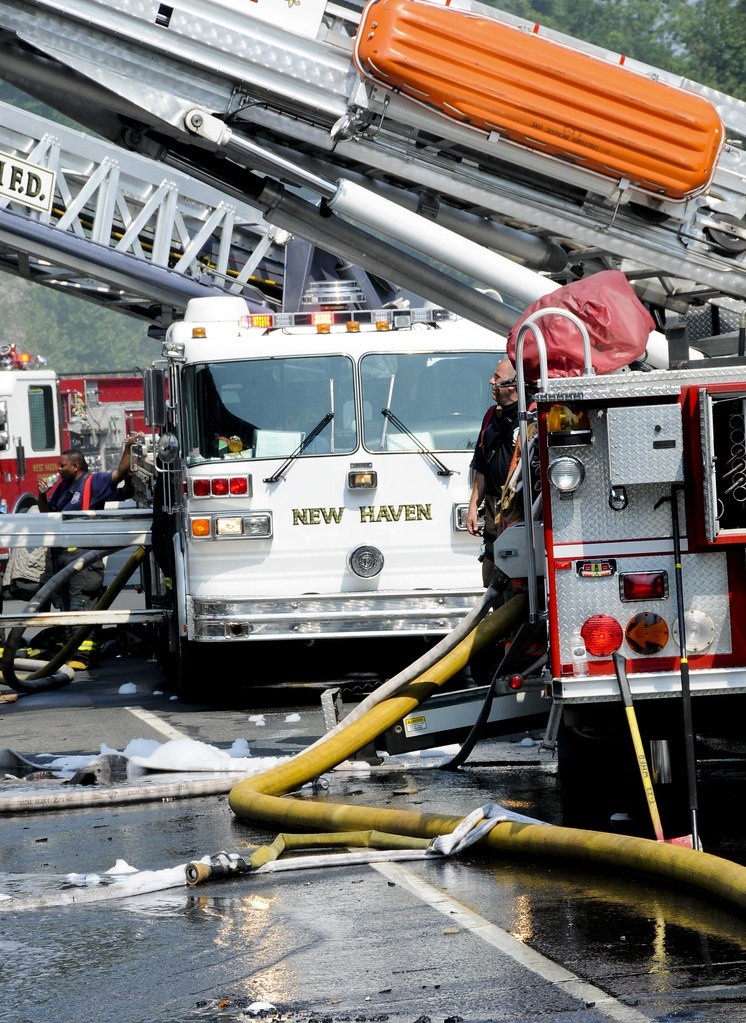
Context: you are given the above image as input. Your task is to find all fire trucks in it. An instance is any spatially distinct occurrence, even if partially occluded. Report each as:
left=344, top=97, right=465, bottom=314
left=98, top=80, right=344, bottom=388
left=146, top=295, right=520, bottom=689
left=0, top=368, right=160, bottom=515
left=514, top=302, right=746, bottom=826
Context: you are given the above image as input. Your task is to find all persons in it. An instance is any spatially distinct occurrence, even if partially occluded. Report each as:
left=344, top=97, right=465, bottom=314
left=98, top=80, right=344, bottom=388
left=35, top=430, right=143, bottom=665
left=465, top=357, right=537, bottom=613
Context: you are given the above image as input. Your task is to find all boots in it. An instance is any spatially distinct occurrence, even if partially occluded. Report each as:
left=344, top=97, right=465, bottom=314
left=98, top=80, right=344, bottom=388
left=65, top=586, right=107, bottom=673
